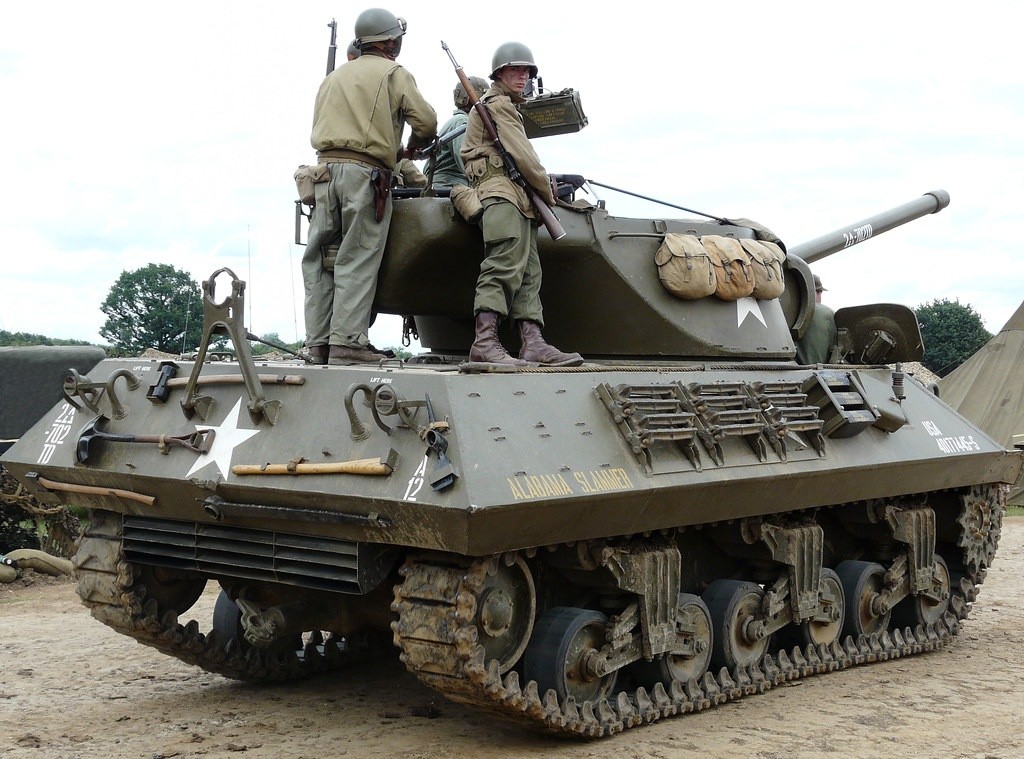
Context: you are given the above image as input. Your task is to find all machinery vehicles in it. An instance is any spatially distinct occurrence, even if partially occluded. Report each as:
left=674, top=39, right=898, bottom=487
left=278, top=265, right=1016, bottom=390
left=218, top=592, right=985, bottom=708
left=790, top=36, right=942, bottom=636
left=0, top=172, right=1024, bottom=741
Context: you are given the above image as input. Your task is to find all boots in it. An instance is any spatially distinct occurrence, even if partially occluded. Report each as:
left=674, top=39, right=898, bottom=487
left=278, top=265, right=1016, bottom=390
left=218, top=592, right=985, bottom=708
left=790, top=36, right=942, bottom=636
left=518, top=318, right=584, bottom=368
left=470, top=310, right=528, bottom=367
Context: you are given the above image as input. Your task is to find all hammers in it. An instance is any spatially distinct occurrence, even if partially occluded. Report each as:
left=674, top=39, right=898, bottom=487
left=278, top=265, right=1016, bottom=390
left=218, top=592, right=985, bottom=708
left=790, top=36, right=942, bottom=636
left=153, top=363, right=307, bottom=407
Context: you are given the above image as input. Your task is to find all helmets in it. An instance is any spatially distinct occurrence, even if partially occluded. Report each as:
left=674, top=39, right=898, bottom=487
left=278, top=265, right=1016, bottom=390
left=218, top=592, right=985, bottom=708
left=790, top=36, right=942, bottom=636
left=454, top=76, right=491, bottom=112
left=346, top=39, right=361, bottom=59
left=489, top=42, right=538, bottom=79
left=353, top=8, right=407, bottom=49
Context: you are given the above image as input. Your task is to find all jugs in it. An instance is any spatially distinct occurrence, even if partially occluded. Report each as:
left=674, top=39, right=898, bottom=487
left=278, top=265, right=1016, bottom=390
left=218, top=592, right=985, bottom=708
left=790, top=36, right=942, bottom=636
left=294, top=165, right=314, bottom=205
left=449, top=183, right=483, bottom=223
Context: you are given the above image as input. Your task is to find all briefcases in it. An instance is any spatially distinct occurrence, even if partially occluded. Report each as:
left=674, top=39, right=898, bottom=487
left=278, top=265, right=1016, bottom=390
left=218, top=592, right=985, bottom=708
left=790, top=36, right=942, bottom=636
left=514, top=87, right=589, bottom=140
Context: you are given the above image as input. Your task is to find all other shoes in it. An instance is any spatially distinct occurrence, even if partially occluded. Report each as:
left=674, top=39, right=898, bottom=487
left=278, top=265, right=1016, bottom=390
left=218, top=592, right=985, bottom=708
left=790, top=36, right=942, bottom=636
left=304, top=346, right=329, bottom=364
left=328, top=345, right=388, bottom=366
left=367, top=340, right=398, bottom=358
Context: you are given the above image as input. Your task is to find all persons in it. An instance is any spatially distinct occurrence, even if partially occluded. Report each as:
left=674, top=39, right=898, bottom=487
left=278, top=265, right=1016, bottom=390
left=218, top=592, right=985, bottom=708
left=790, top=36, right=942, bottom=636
left=460, top=43, right=585, bottom=367
left=347, top=39, right=490, bottom=197
left=793, top=274, right=837, bottom=365
left=300, top=8, right=438, bottom=364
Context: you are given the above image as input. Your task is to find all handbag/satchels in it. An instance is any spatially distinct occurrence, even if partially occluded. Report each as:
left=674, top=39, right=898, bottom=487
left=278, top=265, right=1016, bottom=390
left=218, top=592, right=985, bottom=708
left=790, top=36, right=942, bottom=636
left=293, top=165, right=316, bottom=207
left=449, top=182, right=484, bottom=224
left=699, top=233, right=755, bottom=300
left=736, top=237, right=786, bottom=300
left=655, top=232, right=716, bottom=299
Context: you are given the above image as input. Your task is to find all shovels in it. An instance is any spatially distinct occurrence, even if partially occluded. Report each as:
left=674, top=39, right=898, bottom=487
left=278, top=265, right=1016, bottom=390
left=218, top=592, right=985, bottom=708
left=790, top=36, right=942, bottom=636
left=73, top=415, right=217, bottom=465
left=425, top=394, right=454, bottom=493
